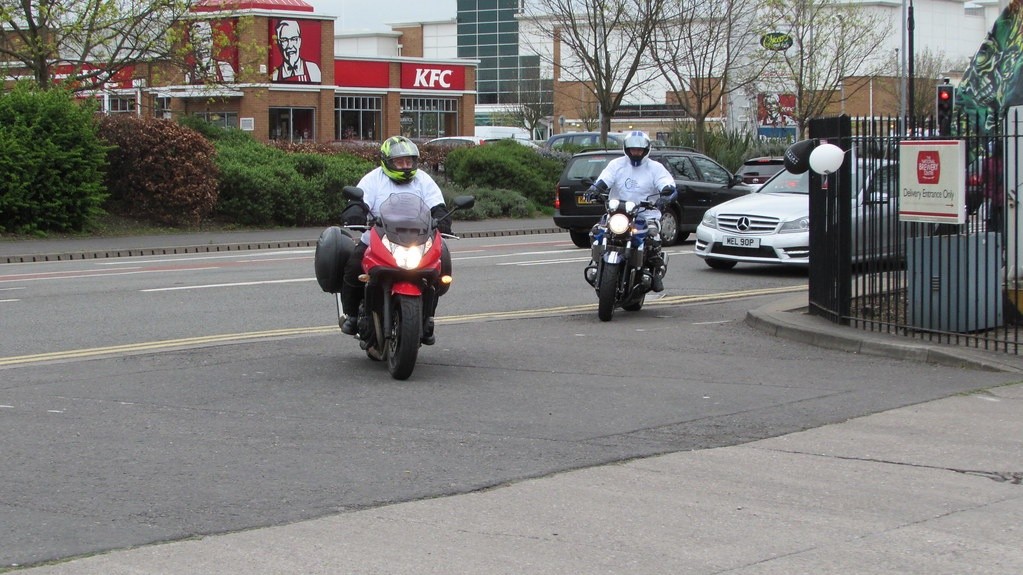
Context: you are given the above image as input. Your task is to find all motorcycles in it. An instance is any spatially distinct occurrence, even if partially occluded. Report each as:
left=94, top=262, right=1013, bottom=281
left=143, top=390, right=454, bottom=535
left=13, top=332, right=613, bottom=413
left=314, top=186, right=475, bottom=380
left=580, top=175, right=675, bottom=321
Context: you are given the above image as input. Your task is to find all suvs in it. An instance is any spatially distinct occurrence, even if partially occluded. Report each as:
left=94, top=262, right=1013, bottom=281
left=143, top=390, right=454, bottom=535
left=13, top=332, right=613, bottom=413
left=552, top=146, right=751, bottom=249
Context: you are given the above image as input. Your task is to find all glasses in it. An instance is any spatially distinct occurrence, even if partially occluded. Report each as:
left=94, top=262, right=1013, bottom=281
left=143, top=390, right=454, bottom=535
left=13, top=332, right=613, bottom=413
left=392, top=158, right=414, bottom=166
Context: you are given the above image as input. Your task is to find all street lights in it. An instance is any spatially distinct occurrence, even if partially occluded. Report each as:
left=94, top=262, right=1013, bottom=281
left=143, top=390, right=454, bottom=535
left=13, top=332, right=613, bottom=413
left=837, top=13, right=845, bottom=113
left=895, top=47, right=898, bottom=114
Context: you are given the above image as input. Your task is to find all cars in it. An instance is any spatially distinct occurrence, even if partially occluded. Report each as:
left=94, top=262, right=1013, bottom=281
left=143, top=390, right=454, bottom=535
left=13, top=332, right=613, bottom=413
left=695, top=154, right=958, bottom=271
left=734, top=154, right=809, bottom=187
left=544, top=134, right=663, bottom=149
left=967, top=157, right=987, bottom=207
left=425, top=138, right=540, bottom=152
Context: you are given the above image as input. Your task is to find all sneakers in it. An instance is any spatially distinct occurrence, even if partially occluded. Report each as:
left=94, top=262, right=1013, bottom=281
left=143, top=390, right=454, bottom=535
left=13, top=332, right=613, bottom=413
left=651, top=274, right=665, bottom=292
left=589, top=268, right=597, bottom=283
left=341, top=315, right=358, bottom=335
left=422, top=334, right=435, bottom=345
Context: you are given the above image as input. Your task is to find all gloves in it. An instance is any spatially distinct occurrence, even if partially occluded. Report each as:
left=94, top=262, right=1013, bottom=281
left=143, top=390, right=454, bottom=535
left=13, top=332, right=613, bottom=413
left=341, top=200, right=370, bottom=233
left=655, top=185, right=681, bottom=212
left=582, top=179, right=608, bottom=202
left=430, top=203, right=453, bottom=239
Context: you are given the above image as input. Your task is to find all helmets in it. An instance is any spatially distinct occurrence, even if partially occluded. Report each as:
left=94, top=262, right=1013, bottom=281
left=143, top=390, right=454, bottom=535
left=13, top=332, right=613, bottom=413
left=379, top=135, right=420, bottom=184
left=622, top=130, right=654, bottom=166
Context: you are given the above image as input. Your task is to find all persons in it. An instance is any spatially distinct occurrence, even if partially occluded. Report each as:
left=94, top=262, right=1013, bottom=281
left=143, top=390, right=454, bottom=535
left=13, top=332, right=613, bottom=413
left=583, top=131, right=677, bottom=293
left=400, top=124, right=415, bottom=137
left=271, top=128, right=276, bottom=139
left=277, top=127, right=288, bottom=138
left=341, top=136, right=452, bottom=345
left=342, top=126, right=357, bottom=139
left=295, top=128, right=311, bottom=139
left=984, top=139, right=1005, bottom=266
left=368, top=129, right=373, bottom=138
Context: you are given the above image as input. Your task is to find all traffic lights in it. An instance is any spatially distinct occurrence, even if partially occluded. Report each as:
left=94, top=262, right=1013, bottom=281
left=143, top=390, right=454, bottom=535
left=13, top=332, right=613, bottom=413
left=937, top=85, right=954, bottom=130
